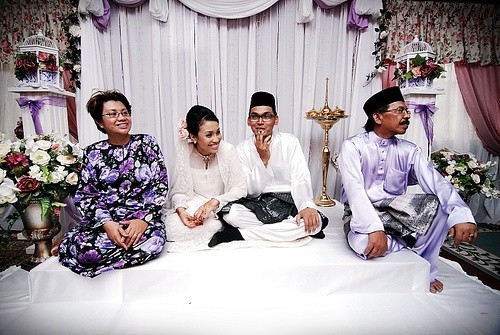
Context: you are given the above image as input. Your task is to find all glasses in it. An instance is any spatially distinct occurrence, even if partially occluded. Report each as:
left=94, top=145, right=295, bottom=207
left=249, top=113, right=276, bottom=119
left=102, top=111, right=130, bottom=118
left=378, top=109, right=412, bottom=116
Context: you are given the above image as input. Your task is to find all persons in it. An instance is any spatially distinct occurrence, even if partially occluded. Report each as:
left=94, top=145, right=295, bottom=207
left=161, top=105, right=235, bottom=242
left=58, top=92, right=169, bottom=278
left=208, top=92, right=328, bottom=248
left=338, top=86, right=477, bottom=293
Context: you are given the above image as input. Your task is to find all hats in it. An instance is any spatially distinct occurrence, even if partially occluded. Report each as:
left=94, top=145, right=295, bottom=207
left=249, top=91, right=278, bottom=116
left=363, top=86, right=405, bottom=118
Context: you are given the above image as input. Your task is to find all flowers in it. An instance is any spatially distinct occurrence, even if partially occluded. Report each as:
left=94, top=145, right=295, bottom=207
left=176, top=119, right=189, bottom=141
left=362, top=0, right=389, bottom=87
left=431, top=148, right=500, bottom=199
left=0, top=0, right=81, bottom=93
left=0, top=132, right=83, bottom=240
left=391, top=54, right=448, bottom=82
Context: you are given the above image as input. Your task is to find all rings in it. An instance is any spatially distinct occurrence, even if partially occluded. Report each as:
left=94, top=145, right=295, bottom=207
left=469, top=235, right=474, bottom=240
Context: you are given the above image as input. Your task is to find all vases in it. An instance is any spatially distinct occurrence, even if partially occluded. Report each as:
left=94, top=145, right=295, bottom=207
left=12, top=197, right=61, bottom=264
